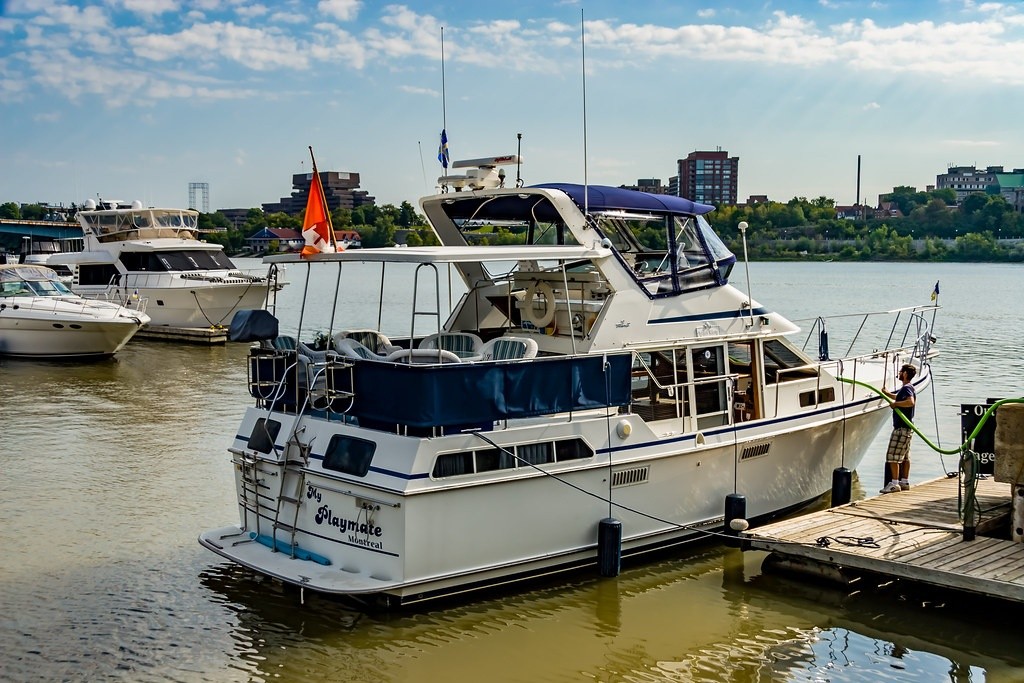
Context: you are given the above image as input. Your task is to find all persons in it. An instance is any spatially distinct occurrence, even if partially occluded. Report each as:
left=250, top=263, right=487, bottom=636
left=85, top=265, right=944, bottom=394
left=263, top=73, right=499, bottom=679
left=879, top=364, right=917, bottom=493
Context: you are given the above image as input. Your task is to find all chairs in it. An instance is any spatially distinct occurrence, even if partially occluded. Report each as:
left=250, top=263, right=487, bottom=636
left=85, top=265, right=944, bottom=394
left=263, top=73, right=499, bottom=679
left=272, top=336, right=339, bottom=391
left=418, top=332, right=483, bottom=353
left=477, top=337, right=538, bottom=363
left=386, top=348, right=461, bottom=365
left=338, top=338, right=388, bottom=362
left=334, top=329, right=403, bottom=356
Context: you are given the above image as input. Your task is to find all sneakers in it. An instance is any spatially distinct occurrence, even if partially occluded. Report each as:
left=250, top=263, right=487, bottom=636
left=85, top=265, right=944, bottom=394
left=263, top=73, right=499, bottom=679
left=879, top=482, right=902, bottom=493
left=898, top=481, right=910, bottom=490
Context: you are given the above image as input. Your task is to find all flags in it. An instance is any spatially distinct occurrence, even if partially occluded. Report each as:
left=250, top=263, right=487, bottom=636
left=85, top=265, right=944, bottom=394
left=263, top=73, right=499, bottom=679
left=931, top=281, right=940, bottom=300
left=301, top=168, right=330, bottom=255
left=437, top=129, right=450, bottom=168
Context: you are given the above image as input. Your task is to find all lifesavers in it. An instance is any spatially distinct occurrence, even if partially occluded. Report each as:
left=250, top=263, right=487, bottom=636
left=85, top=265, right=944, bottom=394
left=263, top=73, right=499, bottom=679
left=524, top=282, right=556, bottom=328
